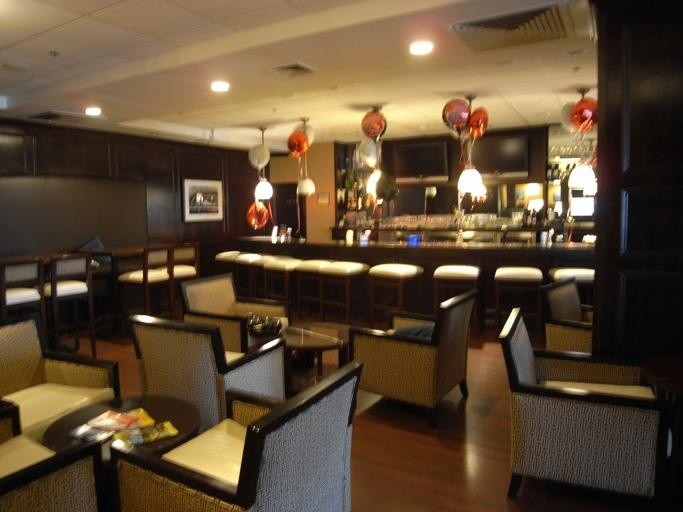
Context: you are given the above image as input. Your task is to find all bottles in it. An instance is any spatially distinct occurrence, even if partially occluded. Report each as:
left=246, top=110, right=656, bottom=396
left=472, top=196, right=484, bottom=209
left=337, top=181, right=371, bottom=211
left=521, top=209, right=546, bottom=226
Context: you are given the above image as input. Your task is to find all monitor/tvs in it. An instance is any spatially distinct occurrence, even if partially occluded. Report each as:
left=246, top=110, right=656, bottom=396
left=471, top=134, right=528, bottom=179
left=395, top=141, right=449, bottom=183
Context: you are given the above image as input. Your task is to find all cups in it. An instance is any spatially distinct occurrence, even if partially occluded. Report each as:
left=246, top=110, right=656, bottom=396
left=406, top=235, right=419, bottom=244
left=358, top=211, right=522, bottom=226
left=550, top=143, right=587, bottom=156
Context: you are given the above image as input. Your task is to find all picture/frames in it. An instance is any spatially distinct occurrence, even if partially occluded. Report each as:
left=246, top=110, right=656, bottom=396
left=181, top=178, right=224, bottom=223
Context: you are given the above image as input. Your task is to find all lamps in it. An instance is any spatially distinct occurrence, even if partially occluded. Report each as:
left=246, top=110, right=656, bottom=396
left=457, top=94, right=482, bottom=192
left=254, top=124, right=274, bottom=201
left=295, top=116, right=316, bottom=195
left=566, top=87, right=596, bottom=190
left=365, top=106, right=383, bottom=195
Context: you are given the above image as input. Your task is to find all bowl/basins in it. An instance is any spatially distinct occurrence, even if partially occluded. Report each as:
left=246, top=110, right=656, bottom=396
left=247, top=315, right=281, bottom=338
